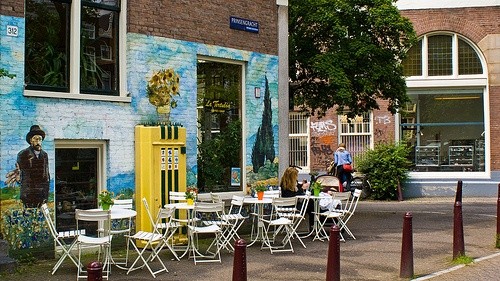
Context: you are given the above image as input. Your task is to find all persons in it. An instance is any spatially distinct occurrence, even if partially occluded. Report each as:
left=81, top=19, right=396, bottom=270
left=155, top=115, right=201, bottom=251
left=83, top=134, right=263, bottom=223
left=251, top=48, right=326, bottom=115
left=281, top=167, right=326, bottom=234
left=333, top=143, right=353, bottom=198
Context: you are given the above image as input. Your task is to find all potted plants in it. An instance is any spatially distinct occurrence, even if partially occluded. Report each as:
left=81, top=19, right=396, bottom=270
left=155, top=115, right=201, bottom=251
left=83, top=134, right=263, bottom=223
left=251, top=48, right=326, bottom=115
left=198, top=134, right=225, bottom=191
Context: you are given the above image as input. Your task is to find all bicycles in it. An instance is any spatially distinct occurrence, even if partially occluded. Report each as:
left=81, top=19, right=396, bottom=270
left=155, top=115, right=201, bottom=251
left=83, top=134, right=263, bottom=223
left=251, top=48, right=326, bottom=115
left=327, top=167, right=372, bottom=201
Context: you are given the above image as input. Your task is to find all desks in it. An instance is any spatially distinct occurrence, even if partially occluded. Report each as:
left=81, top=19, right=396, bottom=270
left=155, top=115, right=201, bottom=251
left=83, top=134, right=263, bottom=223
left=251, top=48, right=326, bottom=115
left=163, top=203, right=219, bottom=260
left=91, top=209, right=137, bottom=270
left=243, top=198, right=278, bottom=249
left=296, top=195, right=332, bottom=239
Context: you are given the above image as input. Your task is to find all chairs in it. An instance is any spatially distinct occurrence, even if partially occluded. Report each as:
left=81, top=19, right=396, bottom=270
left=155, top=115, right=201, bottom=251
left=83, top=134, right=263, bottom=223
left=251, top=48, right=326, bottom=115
left=42, top=189, right=363, bottom=281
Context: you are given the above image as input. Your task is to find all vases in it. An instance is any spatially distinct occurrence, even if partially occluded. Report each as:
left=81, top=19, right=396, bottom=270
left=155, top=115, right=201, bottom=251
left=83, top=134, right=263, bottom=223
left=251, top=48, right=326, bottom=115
left=103, top=205, right=110, bottom=210
left=258, top=192, right=264, bottom=200
left=313, top=189, right=320, bottom=197
left=187, top=198, right=195, bottom=204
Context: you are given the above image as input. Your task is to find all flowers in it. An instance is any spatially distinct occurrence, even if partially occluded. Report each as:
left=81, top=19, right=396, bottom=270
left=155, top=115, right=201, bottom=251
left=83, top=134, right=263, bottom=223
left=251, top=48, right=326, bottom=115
left=97, top=190, right=114, bottom=206
left=311, top=180, right=322, bottom=189
left=185, top=186, right=198, bottom=201
left=255, top=181, right=266, bottom=192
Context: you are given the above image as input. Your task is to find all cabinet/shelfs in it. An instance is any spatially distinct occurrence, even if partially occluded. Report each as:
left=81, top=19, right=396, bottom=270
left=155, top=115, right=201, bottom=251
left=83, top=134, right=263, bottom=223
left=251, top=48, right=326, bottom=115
left=416, top=146, right=440, bottom=167
left=475, top=140, right=485, bottom=172
left=448, top=146, right=474, bottom=166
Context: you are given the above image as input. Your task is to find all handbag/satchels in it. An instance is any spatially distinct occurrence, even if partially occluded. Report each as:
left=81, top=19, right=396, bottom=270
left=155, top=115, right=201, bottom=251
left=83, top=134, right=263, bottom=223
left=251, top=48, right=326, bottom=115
left=343, top=165, right=351, bottom=171
